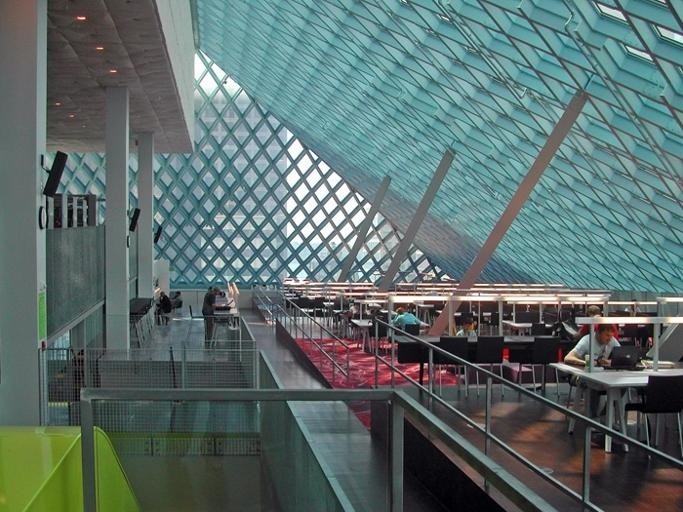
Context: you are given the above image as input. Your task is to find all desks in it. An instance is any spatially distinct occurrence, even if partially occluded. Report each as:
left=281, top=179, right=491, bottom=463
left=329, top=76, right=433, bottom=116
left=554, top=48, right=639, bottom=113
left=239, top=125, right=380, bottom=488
left=394, top=336, right=575, bottom=392
left=548, top=361, right=683, bottom=453
left=206, top=288, right=242, bottom=350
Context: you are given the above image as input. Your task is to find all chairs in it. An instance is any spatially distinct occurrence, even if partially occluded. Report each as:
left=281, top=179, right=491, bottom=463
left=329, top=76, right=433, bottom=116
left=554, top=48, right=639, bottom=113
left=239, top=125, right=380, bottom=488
left=129, top=298, right=155, bottom=349
left=624, top=375, right=683, bottom=459
left=404, top=312, right=560, bottom=398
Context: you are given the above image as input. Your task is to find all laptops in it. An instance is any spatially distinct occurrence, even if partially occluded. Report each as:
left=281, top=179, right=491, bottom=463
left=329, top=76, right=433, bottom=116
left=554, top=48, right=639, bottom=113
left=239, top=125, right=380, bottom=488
left=594, top=346, right=639, bottom=370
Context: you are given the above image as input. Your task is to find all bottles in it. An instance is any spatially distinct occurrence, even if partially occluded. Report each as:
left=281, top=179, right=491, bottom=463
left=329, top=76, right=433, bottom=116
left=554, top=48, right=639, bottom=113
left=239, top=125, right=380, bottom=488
left=585, top=355, right=590, bottom=373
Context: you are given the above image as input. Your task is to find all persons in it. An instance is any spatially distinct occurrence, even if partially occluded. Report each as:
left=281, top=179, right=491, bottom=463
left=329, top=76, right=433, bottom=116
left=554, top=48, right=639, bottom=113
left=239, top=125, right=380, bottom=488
left=171, top=291, right=182, bottom=309
left=334, top=289, right=349, bottom=310
left=351, top=303, right=368, bottom=327
left=391, top=306, right=421, bottom=327
left=156, top=291, right=172, bottom=313
left=623, top=299, right=641, bottom=312
left=456, top=316, right=478, bottom=336
left=202, top=286, right=221, bottom=347
left=563, top=324, right=626, bottom=415
left=575, top=306, right=619, bottom=341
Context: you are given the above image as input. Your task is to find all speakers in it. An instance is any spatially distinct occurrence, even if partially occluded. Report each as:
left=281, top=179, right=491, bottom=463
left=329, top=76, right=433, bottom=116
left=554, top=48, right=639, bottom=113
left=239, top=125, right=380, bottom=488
left=43, top=151, right=68, bottom=196
left=130, top=208, right=141, bottom=232
left=154, top=226, right=163, bottom=243
